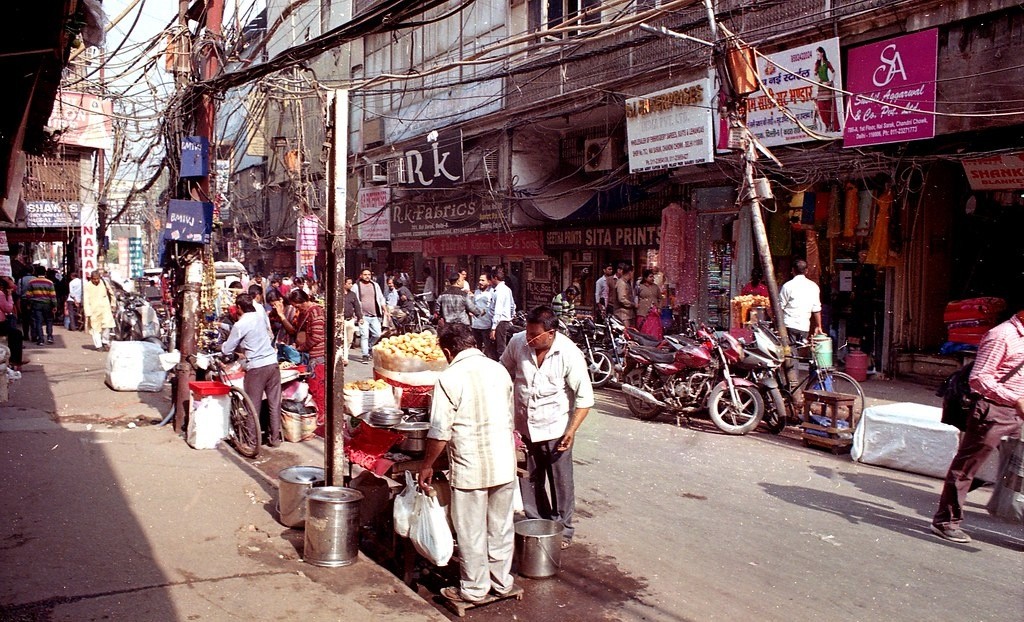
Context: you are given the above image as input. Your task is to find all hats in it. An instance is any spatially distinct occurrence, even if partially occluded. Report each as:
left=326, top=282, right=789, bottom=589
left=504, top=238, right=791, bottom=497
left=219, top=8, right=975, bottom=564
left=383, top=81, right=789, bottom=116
left=236, top=294, right=257, bottom=313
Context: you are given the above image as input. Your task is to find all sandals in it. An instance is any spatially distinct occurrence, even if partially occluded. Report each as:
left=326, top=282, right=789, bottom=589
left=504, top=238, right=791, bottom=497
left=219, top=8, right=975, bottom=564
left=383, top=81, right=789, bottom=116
left=440, top=588, right=486, bottom=604
left=489, top=587, right=508, bottom=598
left=561, top=542, right=570, bottom=548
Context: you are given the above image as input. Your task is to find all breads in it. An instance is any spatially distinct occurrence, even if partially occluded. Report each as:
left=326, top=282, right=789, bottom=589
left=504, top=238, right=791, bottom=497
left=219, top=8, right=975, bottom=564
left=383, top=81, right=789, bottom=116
left=732, top=294, right=772, bottom=306
left=377, top=330, right=447, bottom=361
left=343, top=379, right=389, bottom=391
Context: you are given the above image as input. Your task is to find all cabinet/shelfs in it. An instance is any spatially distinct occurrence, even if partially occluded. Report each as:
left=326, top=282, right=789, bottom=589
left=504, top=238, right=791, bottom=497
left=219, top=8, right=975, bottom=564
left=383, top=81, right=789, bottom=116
left=708, top=239, right=735, bottom=330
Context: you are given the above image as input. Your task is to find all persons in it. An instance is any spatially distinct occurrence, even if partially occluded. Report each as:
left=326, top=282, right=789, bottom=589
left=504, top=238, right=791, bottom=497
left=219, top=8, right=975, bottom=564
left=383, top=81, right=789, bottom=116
left=499, top=305, right=595, bottom=550
left=215, top=281, right=325, bottom=446
left=778, top=260, right=827, bottom=383
left=633, top=266, right=666, bottom=341
left=423, top=266, right=438, bottom=315
left=418, top=323, right=517, bottom=603
left=67, top=273, right=82, bottom=331
left=376, top=268, right=415, bottom=305
left=742, top=268, right=773, bottom=319
left=496, top=265, right=514, bottom=292
left=255, top=270, right=325, bottom=326
left=458, top=268, right=470, bottom=291
left=146, top=281, right=161, bottom=301
left=434, top=272, right=486, bottom=334
left=344, top=276, right=364, bottom=366
left=484, top=265, right=494, bottom=278
left=930, top=308, right=1024, bottom=542
left=490, top=271, right=516, bottom=360
left=83, top=270, right=117, bottom=351
left=814, top=45, right=841, bottom=132
left=351, top=269, right=387, bottom=364
left=0, top=260, right=58, bottom=365
left=596, top=263, right=640, bottom=327
left=469, top=273, right=499, bottom=361
left=550, top=285, right=580, bottom=323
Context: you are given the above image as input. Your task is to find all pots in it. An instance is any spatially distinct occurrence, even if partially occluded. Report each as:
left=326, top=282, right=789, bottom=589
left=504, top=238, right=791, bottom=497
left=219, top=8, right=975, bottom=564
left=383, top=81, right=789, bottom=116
left=371, top=409, right=405, bottom=425
left=390, top=422, right=430, bottom=452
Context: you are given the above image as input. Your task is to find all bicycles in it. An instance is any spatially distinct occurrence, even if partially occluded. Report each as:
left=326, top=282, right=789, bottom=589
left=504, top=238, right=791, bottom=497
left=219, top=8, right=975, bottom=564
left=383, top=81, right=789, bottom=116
left=166, top=351, right=261, bottom=458
left=758, top=324, right=866, bottom=430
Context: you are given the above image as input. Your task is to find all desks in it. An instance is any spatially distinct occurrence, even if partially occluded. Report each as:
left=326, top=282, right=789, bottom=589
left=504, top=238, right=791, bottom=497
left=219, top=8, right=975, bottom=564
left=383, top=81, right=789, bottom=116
left=310, top=425, right=523, bottom=562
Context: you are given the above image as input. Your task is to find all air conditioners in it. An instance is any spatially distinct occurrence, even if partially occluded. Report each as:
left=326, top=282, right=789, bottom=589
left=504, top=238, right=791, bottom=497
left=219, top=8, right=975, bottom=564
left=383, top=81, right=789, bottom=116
left=364, top=164, right=387, bottom=182
left=584, top=137, right=622, bottom=173
left=309, top=188, right=320, bottom=208
left=463, top=148, right=498, bottom=184
left=387, top=157, right=409, bottom=185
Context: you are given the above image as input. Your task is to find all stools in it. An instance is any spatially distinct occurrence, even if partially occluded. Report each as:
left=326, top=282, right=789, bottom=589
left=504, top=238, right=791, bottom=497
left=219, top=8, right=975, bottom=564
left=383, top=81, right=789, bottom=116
left=803, top=390, right=858, bottom=456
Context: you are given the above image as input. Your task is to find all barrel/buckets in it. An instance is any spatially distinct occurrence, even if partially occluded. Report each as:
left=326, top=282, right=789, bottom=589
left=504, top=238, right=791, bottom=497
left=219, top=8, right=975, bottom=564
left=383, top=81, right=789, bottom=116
left=349, top=471, right=389, bottom=527
left=276, top=467, right=324, bottom=528
left=811, top=334, right=832, bottom=368
left=514, top=519, right=565, bottom=578
left=187, top=390, right=233, bottom=449
left=843, top=349, right=867, bottom=382
left=303, top=485, right=364, bottom=570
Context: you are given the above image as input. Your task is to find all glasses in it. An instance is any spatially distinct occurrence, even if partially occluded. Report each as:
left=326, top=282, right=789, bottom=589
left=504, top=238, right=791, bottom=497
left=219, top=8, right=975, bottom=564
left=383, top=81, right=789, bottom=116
left=363, top=274, right=371, bottom=276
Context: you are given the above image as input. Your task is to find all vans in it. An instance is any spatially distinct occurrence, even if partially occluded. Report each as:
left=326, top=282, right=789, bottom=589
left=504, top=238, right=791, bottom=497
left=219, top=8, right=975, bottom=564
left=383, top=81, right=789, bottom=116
left=214, top=262, right=248, bottom=286
left=143, top=268, right=163, bottom=281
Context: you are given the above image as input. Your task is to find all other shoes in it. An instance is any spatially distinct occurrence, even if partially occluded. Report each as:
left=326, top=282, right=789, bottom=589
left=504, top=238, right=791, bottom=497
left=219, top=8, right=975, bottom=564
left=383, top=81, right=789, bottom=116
left=31, top=338, right=39, bottom=342
left=22, top=358, right=30, bottom=365
left=15, top=365, right=22, bottom=373
left=97, top=347, right=102, bottom=351
left=102, top=343, right=110, bottom=351
left=22, top=335, right=30, bottom=342
left=47, top=336, right=55, bottom=344
left=39, top=339, right=44, bottom=346
left=362, top=356, right=368, bottom=364
left=930, top=523, right=972, bottom=542
left=343, top=361, right=348, bottom=366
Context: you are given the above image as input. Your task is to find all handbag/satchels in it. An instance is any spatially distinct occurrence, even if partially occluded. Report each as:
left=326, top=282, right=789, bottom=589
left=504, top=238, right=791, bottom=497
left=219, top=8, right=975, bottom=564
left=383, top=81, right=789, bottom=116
left=936, top=360, right=977, bottom=432
left=392, top=470, right=454, bottom=567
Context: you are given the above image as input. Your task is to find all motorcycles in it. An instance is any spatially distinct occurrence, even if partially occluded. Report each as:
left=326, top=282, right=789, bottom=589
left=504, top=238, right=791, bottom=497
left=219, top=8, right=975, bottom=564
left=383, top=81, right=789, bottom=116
left=111, top=289, right=172, bottom=349
left=394, top=291, right=438, bottom=335
left=610, top=318, right=786, bottom=435
left=506, top=308, right=630, bottom=389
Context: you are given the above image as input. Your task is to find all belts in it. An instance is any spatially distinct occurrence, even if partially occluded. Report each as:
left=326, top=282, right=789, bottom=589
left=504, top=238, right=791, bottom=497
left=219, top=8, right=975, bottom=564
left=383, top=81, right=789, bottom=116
left=344, top=317, right=352, bottom=321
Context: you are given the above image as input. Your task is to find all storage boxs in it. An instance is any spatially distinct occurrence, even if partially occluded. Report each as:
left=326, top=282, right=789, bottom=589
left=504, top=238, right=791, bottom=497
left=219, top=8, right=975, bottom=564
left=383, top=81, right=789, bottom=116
left=859, top=402, right=1009, bottom=483
